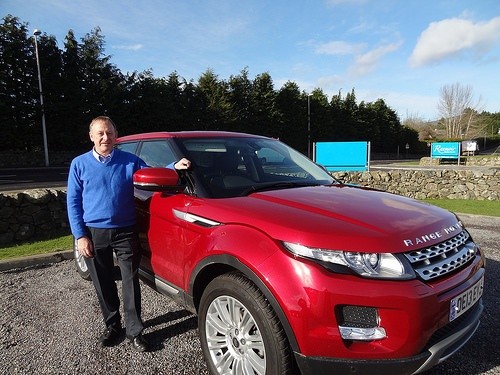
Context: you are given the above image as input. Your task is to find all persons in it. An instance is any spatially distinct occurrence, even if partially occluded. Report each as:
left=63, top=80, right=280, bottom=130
left=67, top=116, right=191, bottom=352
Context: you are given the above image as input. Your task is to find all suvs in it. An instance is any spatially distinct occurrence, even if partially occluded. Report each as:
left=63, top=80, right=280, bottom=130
left=72, top=130, right=486, bottom=375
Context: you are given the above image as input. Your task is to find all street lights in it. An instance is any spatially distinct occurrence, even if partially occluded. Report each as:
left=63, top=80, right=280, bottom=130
left=32, top=28, right=50, bottom=167
left=307, top=94, right=313, bottom=159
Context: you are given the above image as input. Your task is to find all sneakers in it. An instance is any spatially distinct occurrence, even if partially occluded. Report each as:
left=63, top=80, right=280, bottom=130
left=102, top=326, right=118, bottom=344
left=132, top=334, right=150, bottom=352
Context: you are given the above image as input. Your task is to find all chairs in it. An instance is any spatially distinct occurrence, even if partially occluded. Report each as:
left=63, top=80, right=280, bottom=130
left=216, top=153, right=258, bottom=188
